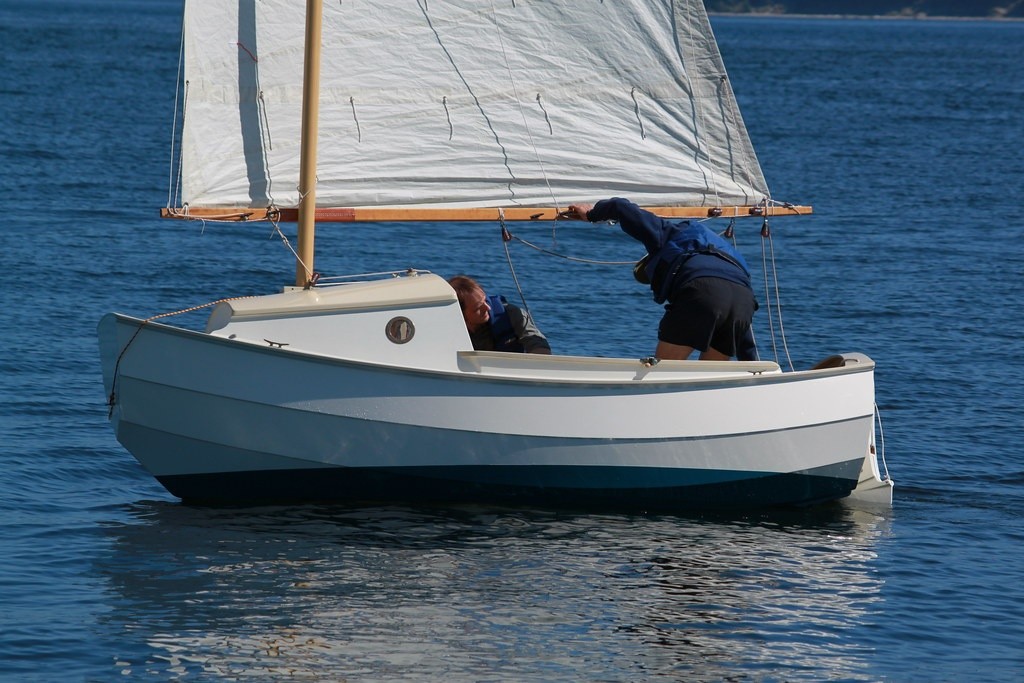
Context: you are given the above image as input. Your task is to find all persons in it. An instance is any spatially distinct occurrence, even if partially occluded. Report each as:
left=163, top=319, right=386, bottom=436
left=568, top=197, right=760, bottom=360
left=448, top=276, right=552, bottom=355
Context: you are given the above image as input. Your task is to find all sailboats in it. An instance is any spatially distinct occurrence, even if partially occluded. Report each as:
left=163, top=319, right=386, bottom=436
left=96, top=0, right=898, bottom=512
